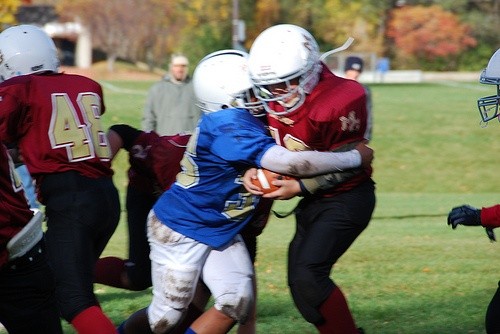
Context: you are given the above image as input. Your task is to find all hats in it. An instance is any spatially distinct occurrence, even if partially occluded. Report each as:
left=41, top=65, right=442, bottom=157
left=345, top=56, right=364, bottom=73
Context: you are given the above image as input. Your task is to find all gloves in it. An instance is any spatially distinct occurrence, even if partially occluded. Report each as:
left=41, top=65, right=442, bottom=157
left=446, top=205, right=482, bottom=228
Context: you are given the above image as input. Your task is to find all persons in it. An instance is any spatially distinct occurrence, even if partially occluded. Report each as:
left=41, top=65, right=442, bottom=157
left=247, top=23, right=376, bottom=334
left=340, top=55, right=374, bottom=143
left=447, top=46, right=500, bottom=334
left=0, top=143, right=64, bottom=334
left=142, top=54, right=201, bottom=137
left=92, top=123, right=273, bottom=334
left=1, top=24, right=121, bottom=334
left=116, top=48, right=373, bottom=334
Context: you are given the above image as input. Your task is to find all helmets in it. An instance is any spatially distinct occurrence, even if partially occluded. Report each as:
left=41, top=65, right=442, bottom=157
left=192, top=50, right=267, bottom=116
left=247, top=24, right=320, bottom=116
left=1, top=24, right=61, bottom=79
left=477, top=48, right=500, bottom=122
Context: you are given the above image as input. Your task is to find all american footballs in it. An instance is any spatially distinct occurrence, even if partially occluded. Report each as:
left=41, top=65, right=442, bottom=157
left=250, top=169, right=296, bottom=194
